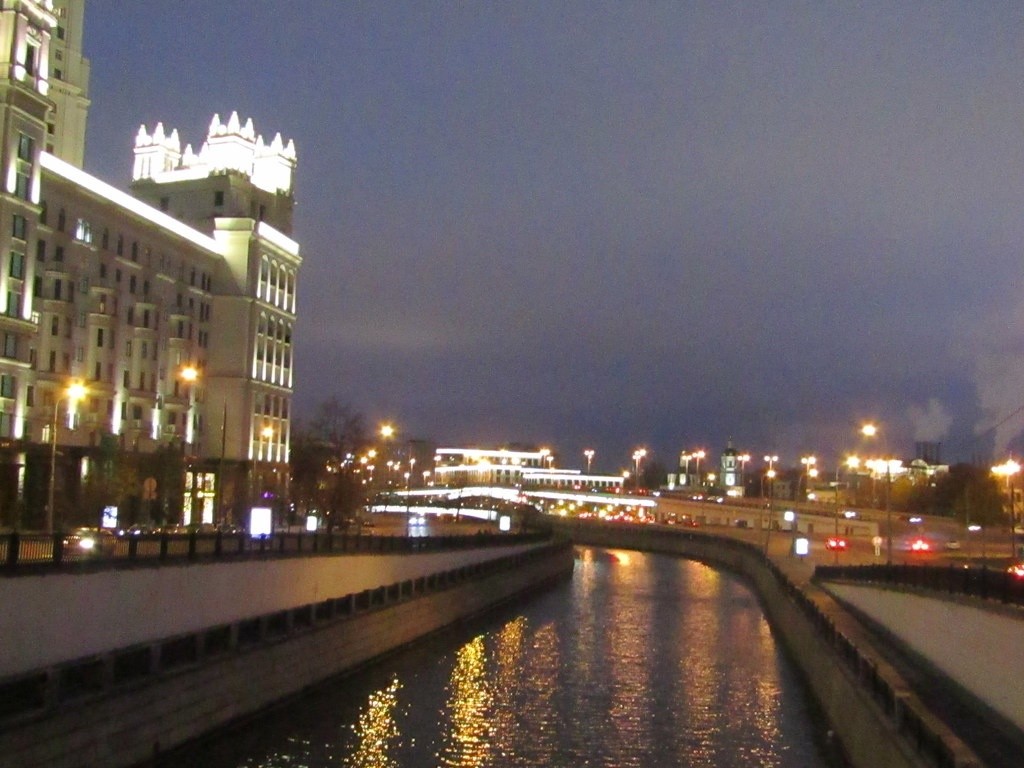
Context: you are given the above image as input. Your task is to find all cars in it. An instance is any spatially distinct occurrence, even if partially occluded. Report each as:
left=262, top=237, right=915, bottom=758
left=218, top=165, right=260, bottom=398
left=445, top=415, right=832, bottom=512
left=826, top=538, right=848, bottom=550
left=944, top=540, right=962, bottom=551
left=62, top=523, right=250, bottom=561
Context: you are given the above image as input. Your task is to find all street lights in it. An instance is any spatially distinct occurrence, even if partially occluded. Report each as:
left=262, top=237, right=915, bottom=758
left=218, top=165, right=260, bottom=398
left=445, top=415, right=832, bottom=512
left=584, top=451, right=594, bottom=475
left=681, top=451, right=706, bottom=487
left=861, top=426, right=890, bottom=464
left=540, top=450, right=549, bottom=468
left=796, top=470, right=817, bottom=507
left=836, top=457, right=859, bottom=505
left=761, top=470, right=775, bottom=499
left=765, top=456, right=778, bottom=497
left=801, top=457, right=816, bottom=483
left=633, top=449, right=646, bottom=494
left=181, top=368, right=226, bottom=530
left=44, top=385, right=85, bottom=535
left=547, top=456, right=552, bottom=468
left=738, top=455, right=749, bottom=486
left=380, top=426, right=413, bottom=537
